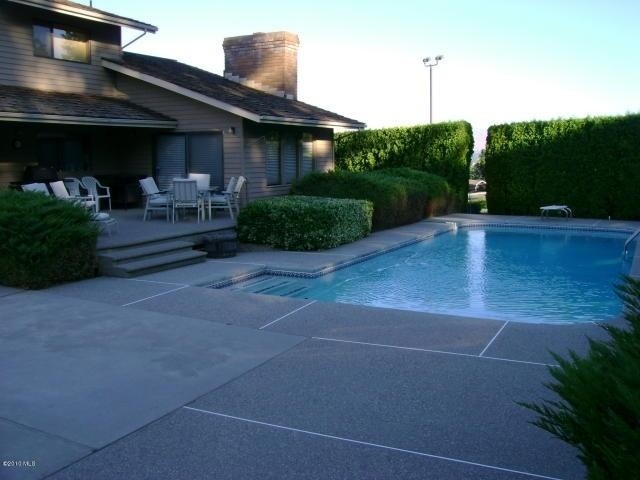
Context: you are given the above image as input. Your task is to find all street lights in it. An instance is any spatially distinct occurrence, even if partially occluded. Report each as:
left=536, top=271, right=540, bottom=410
left=422, top=54, right=441, bottom=124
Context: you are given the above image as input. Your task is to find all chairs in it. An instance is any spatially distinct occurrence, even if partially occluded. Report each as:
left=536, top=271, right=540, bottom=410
left=22, top=176, right=120, bottom=237
left=138, top=172, right=247, bottom=224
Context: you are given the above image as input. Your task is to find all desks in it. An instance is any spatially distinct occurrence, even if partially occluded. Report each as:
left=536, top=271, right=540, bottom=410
left=540, top=204, right=571, bottom=221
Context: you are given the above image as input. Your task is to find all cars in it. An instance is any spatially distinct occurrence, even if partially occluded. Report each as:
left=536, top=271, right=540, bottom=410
left=468, top=178, right=486, bottom=203
